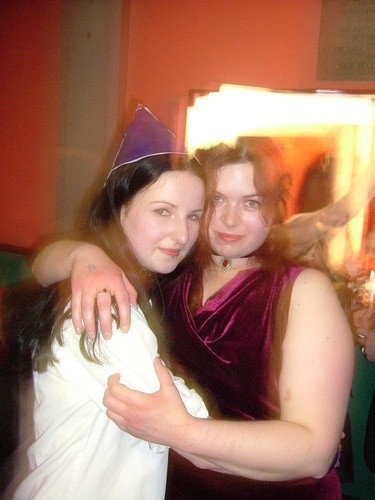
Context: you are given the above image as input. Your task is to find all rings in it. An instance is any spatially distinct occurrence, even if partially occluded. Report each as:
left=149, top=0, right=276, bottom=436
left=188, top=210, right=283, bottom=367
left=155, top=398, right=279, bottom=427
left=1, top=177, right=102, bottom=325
left=361, top=346, right=365, bottom=354
left=95, top=288, right=114, bottom=296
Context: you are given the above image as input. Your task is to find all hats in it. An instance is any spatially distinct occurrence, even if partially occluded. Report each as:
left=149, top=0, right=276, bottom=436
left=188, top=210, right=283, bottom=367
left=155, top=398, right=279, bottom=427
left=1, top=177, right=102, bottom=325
left=107, top=102, right=191, bottom=182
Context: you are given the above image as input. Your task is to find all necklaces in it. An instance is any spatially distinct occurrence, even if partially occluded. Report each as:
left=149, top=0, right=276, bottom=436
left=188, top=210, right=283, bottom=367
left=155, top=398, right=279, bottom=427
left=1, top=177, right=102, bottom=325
left=205, top=254, right=258, bottom=271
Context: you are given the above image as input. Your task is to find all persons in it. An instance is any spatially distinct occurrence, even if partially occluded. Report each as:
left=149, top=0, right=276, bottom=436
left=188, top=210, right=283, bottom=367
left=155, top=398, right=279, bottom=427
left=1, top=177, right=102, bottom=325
left=351, top=287, right=375, bottom=478
left=238, top=135, right=375, bottom=271
left=1, top=145, right=217, bottom=500
left=32, top=144, right=355, bottom=500
